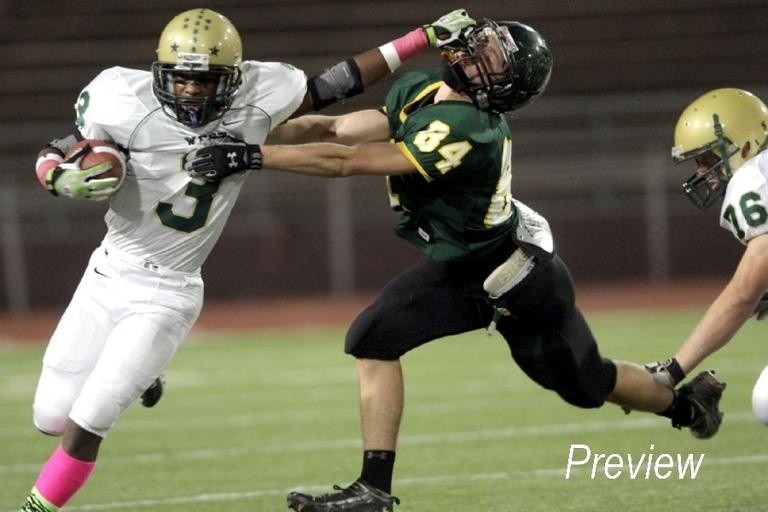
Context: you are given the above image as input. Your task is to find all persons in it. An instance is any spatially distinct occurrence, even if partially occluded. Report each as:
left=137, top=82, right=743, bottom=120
left=184, top=18, right=728, bottom=512
left=623, top=86, right=768, bottom=440
left=20, top=9, right=478, bottom=512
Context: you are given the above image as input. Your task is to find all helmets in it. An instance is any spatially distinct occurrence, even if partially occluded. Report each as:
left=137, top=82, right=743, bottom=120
left=440, top=19, right=552, bottom=112
left=151, top=8, right=243, bottom=127
left=672, top=87, right=768, bottom=213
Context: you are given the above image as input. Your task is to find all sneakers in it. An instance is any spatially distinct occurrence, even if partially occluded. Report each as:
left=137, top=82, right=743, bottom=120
left=287, top=482, right=398, bottom=512
left=679, top=371, right=726, bottom=438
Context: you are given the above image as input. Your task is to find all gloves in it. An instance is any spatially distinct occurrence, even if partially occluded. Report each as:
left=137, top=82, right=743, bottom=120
left=45, top=146, right=118, bottom=202
left=621, top=358, right=686, bottom=415
left=423, top=9, right=477, bottom=48
left=185, top=143, right=262, bottom=182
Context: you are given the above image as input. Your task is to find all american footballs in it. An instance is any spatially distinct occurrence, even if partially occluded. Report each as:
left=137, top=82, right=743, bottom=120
left=62, top=140, right=126, bottom=197
